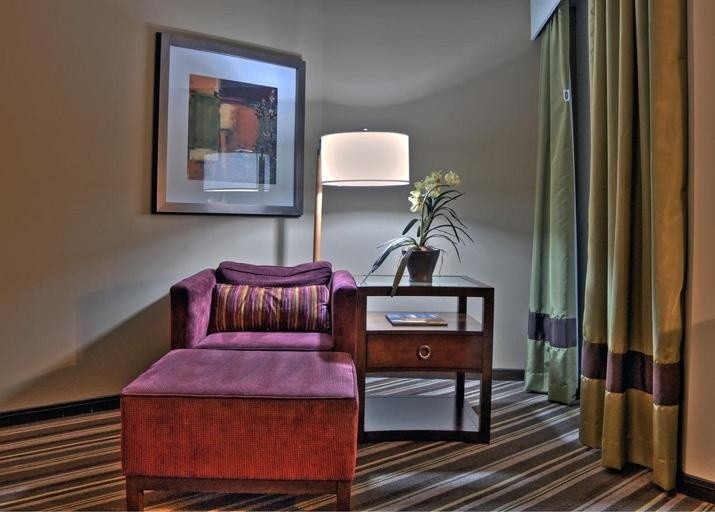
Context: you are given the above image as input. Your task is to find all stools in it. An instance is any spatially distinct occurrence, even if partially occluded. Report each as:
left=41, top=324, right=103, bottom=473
left=120, top=348, right=360, bottom=512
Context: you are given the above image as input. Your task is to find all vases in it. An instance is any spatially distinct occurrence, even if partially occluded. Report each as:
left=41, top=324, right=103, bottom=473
left=402, top=248, right=440, bottom=282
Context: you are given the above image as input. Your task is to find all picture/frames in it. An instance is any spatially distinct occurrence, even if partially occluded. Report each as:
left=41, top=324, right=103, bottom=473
left=150, top=31, right=306, bottom=218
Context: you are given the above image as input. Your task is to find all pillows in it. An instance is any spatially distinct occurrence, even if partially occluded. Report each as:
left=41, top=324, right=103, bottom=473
left=215, top=284, right=330, bottom=333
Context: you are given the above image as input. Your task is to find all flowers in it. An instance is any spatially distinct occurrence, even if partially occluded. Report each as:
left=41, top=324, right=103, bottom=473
left=360, top=170, right=474, bottom=297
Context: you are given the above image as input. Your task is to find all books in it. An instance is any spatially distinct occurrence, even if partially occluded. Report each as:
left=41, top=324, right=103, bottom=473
left=386, top=312, right=448, bottom=326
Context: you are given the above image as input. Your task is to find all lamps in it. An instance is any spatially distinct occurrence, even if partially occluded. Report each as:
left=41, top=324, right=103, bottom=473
left=313, top=129, right=411, bottom=261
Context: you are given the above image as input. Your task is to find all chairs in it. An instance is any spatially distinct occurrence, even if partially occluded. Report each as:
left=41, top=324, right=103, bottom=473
left=120, top=261, right=359, bottom=358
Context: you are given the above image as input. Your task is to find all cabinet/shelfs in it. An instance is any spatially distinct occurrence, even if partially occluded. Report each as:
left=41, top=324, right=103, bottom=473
left=353, top=275, right=494, bottom=445
